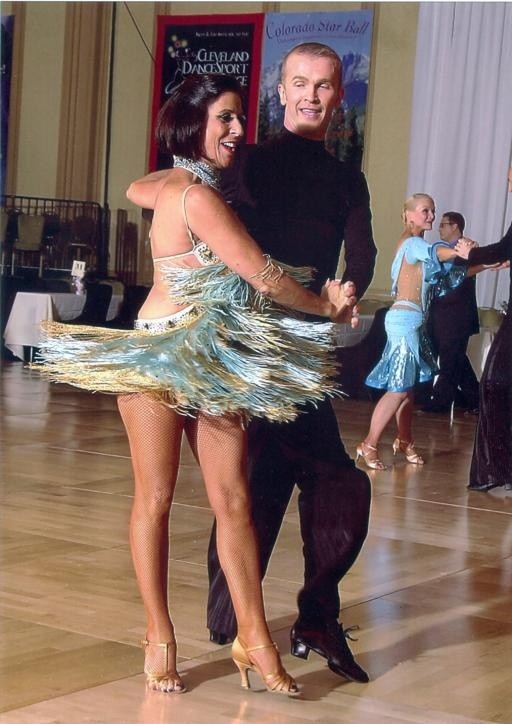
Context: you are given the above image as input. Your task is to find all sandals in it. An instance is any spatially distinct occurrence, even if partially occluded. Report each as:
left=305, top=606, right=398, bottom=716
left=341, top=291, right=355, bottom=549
left=144, top=626, right=188, bottom=694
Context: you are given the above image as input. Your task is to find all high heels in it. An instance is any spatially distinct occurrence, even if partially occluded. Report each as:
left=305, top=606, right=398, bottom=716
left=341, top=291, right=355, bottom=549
left=393, top=439, right=424, bottom=465
left=210, top=629, right=228, bottom=644
left=291, top=615, right=368, bottom=683
left=231, top=636, right=304, bottom=696
left=467, top=474, right=512, bottom=489
left=356, top=441, right=387, bottom=470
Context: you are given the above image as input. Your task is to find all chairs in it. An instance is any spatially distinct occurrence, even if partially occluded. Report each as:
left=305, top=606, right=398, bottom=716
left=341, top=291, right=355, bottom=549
left=1, top=201, right=150, bottom=363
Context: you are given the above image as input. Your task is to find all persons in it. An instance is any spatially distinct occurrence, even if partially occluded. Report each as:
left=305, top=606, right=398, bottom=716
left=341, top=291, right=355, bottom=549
left=202, top=38, right=376, bottom=690
left=452, top=161, right=511, bottom=497
left=420, top=207, right=481, bottom=417
left=348, top=191, right=503, bottom=474
left=118, top=69, right=354, bottom=701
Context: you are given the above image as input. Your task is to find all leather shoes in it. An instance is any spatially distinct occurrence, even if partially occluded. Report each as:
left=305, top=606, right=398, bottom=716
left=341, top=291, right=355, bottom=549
left=419, top=403, right=453, bottom=413
left=463, top=406, right=480, bottom=415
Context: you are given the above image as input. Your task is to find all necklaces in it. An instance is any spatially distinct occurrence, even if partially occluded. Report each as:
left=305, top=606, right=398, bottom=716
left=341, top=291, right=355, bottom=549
left=165, top=152, right=224, bottom=193
